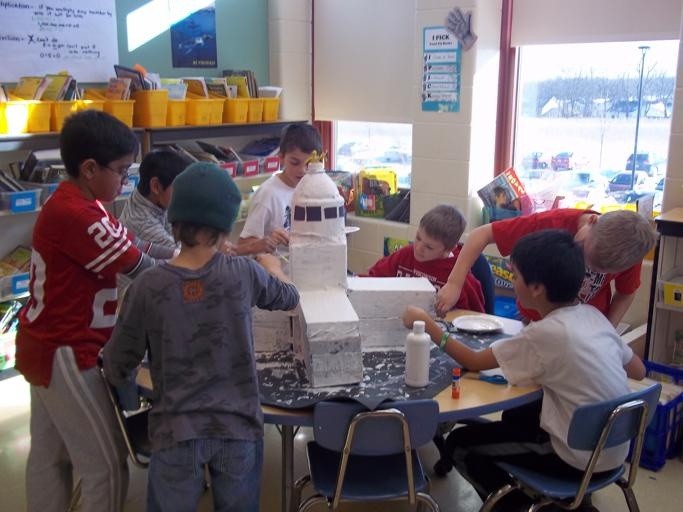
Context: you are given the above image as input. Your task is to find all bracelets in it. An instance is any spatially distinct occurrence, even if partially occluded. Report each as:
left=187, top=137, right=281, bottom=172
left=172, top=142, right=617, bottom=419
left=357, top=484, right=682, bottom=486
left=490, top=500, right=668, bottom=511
left=439, top=332, right=450, bottom=350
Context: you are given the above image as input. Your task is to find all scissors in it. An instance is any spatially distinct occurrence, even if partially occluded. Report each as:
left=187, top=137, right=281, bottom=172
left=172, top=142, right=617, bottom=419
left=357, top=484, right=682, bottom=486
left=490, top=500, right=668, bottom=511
left=466, top=375, right=507, bottom=384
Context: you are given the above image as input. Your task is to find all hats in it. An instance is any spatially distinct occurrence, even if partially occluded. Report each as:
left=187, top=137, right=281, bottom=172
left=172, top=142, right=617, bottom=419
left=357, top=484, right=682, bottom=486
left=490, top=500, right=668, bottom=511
left=163, top=159, right=242, bottom=235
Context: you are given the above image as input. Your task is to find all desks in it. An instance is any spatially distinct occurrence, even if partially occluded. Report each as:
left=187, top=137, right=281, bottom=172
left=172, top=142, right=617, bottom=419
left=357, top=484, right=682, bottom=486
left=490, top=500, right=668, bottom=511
left=120, top=306, right=543, bottom=512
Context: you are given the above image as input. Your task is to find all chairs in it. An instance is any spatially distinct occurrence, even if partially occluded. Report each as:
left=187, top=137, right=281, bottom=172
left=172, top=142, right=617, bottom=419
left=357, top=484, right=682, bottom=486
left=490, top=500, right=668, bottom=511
left=432, top=249, right=497, bottom=479
left=293, top=397, right=441, bottom=512
left=100, top=361, right=212, bottom=499
left=481, top=382, right=662, bottom=512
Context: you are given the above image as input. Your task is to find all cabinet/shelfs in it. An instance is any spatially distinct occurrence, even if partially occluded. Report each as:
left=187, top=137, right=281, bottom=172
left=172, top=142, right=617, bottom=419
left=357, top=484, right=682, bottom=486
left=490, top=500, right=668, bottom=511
left=0, top=118, right=312, bottom=381
left=643, top=207, right=682, bottom=385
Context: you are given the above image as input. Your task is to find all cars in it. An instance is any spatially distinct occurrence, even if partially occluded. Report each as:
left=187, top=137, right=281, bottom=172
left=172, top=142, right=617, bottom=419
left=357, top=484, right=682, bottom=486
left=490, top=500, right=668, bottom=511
left=530, top=145, right=666, bottom=206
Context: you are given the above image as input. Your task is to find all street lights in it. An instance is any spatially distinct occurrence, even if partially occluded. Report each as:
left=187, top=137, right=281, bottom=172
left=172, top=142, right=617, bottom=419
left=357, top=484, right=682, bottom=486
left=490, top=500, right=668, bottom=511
left=629, top=45, right=649, bottom=192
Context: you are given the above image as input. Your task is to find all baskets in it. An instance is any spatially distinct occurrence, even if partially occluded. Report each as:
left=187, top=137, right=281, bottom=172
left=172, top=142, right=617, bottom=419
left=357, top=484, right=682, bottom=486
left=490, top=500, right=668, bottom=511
left=625, top=358, right=683, bottom=472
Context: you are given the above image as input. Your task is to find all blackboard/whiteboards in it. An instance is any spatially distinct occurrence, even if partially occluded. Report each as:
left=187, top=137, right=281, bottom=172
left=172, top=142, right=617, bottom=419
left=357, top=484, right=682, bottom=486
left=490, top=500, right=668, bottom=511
left=0, top=0, right=119, bottom=83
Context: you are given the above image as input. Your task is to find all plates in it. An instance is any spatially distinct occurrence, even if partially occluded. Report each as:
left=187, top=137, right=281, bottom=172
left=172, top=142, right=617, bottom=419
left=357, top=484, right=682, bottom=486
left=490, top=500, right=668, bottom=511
left=452, top=315, right=503, bottom=334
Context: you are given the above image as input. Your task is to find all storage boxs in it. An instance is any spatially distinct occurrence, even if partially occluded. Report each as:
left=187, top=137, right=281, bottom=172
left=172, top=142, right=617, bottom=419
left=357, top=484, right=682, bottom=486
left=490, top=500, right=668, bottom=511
left=0, top=89, right=281, bottom=215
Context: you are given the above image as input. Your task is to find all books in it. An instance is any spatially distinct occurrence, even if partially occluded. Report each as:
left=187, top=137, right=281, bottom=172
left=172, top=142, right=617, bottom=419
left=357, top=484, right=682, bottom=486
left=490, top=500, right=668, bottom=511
left=0, top=147, right=68, bottom=190
left=133, top=126, right=280, bottom=167
left=0, top=244, right=32, bottom=341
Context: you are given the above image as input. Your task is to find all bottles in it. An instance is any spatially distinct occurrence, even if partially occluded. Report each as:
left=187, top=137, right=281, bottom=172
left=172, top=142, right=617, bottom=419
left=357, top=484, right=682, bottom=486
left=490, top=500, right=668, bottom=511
left=404, top=320, right=430, bottom=386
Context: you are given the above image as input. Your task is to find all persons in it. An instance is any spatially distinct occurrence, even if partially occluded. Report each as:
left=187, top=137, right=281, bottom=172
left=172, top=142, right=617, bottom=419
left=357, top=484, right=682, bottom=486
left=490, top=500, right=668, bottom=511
left=239, top=125, right=323, bottom=269
left=494, top=187, right=520, bottom=220
left=368, top=204, right=487, bottom=313
left=102, top=161, right=300, bottom=512
left=116, top=151, right=278, bottom=300
left=13, top=108, right=182, bottom=512
left=436, top=208, right=660, bottom=329
left=401, top=230, right=648, bottom=512
